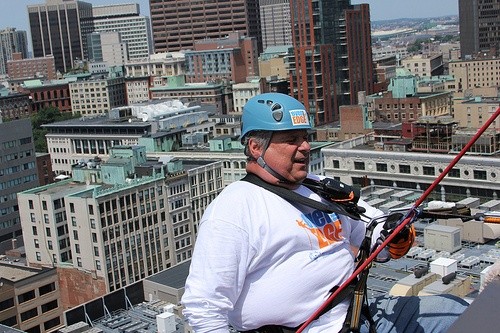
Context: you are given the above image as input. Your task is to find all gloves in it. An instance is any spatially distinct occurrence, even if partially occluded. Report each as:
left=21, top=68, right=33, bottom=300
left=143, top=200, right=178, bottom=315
left=377, top=213, right=416, bottom=259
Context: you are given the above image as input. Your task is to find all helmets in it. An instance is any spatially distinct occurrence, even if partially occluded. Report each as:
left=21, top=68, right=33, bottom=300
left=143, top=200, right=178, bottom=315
left=239, top=92, right=312, bottom=145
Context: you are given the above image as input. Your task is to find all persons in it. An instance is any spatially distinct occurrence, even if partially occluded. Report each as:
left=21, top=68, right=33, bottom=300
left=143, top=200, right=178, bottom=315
left=180, top=90, right=472, bottom=333
